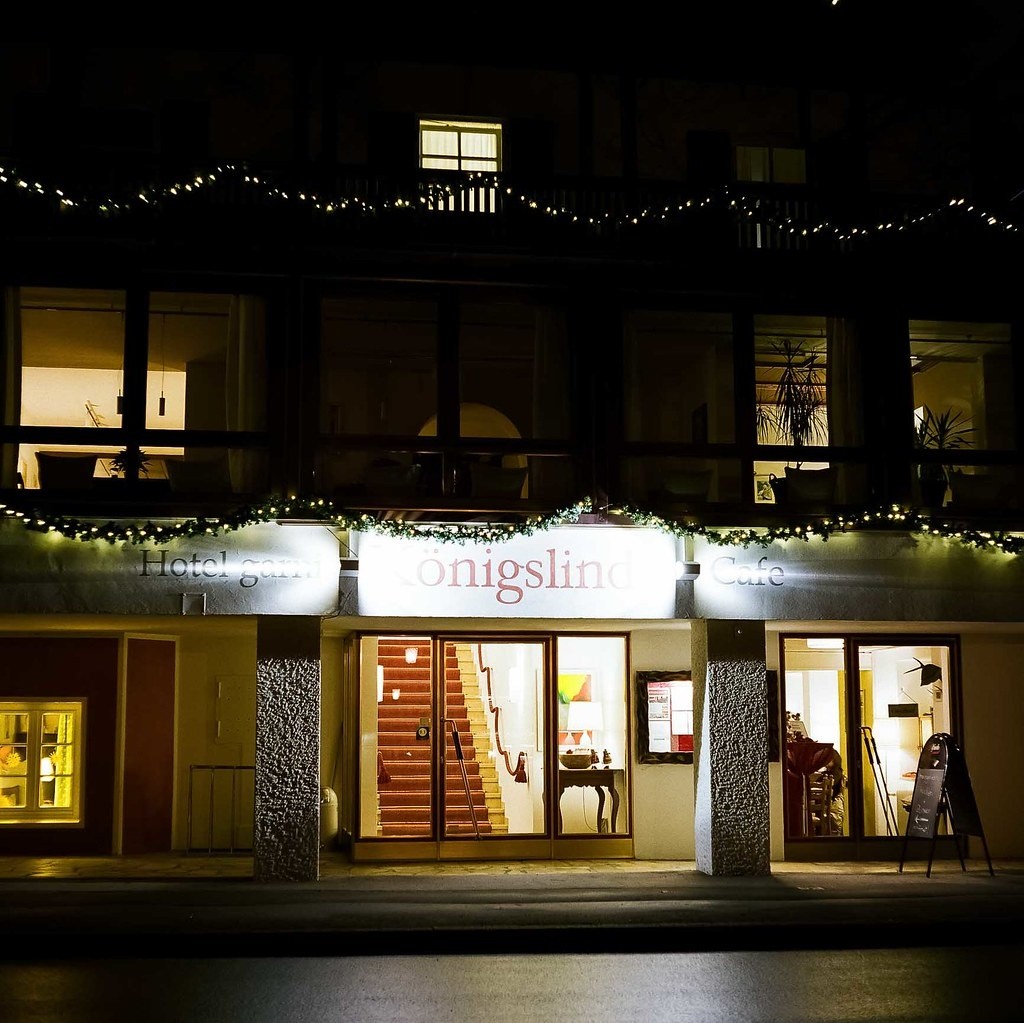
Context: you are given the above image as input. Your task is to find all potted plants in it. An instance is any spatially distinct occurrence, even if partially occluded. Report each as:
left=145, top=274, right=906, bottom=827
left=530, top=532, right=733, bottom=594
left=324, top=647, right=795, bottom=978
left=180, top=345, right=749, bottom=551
left=109, top=446, right=153, bottom=478
left=915, top=401, right=979, bottom=505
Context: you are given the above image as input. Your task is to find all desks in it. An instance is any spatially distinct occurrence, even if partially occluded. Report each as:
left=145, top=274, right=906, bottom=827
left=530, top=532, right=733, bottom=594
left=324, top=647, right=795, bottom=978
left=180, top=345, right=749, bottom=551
left=541, top=769, right=619, bottom=833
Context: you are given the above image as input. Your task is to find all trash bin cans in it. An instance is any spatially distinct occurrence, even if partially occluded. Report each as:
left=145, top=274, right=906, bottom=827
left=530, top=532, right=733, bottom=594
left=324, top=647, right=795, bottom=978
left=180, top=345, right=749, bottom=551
left=320, top=786, right=338, bottom=850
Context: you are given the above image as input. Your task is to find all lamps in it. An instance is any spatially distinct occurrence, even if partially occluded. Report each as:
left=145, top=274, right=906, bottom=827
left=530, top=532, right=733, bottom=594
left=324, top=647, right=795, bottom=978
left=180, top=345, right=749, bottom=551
left=568, top=701, right=601, bottom=748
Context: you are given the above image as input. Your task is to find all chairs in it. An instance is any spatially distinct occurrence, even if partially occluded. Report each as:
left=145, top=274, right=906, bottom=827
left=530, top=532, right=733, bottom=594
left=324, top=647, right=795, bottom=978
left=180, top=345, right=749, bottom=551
left=808, top=772, right=834, bottom=835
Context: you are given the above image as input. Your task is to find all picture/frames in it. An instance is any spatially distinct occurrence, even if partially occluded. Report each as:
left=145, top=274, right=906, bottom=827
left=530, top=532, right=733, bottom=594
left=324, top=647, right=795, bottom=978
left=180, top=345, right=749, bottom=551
left=535, top=667, right=596, bottom=751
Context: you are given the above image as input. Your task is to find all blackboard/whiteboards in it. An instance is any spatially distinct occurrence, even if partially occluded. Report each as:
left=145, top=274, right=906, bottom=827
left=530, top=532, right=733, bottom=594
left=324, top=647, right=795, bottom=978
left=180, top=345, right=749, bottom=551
left=904, top=767, right=947, bottom=844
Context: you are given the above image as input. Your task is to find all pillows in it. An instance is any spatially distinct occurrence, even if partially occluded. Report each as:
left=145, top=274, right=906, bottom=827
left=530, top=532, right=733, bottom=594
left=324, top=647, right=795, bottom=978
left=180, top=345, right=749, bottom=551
left=33, top=451, right=98, bottom=490
left=780, top=466, right=838, bottom=503
